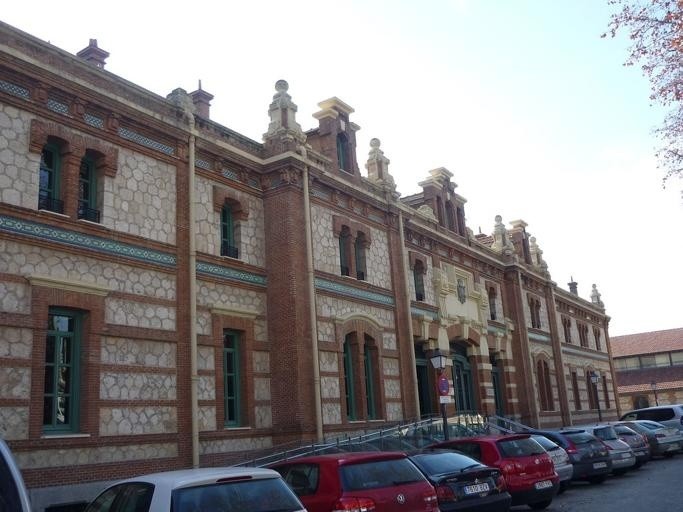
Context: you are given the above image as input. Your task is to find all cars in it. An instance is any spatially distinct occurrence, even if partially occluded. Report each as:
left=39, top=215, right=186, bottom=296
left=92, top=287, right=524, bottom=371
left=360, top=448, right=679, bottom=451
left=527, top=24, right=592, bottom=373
left=412, top=434, right=560, bottom=511
left=583, top=422, right=650, bottom=471
left=514, top=430, right=612, bottom=490
left=385, top=449, right=511, bottom=512
left=264, top=451, right=441, bottom=512
left=0, top=437, right=33, bottom=512
left=605, top=420, right=682, bottom=461
left=558, top=425, right=635, bottom=479
left=83, top=467, right=308, bottom=511
left=530, top=435, right=573, bottom=496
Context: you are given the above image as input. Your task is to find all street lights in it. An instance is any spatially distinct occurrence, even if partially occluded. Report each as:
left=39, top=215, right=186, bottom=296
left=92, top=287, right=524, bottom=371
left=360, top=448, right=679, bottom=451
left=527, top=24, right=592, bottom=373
left=429, top=346, right=449, bottom=441
left=651, top=380, right=659, bottom=406
left=589, top=371, right=602, bottom=422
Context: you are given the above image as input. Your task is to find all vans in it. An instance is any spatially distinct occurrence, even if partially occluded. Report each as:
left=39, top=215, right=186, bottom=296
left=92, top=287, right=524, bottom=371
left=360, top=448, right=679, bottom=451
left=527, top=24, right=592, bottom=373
left=619, top=404, right=682, bottom=432
